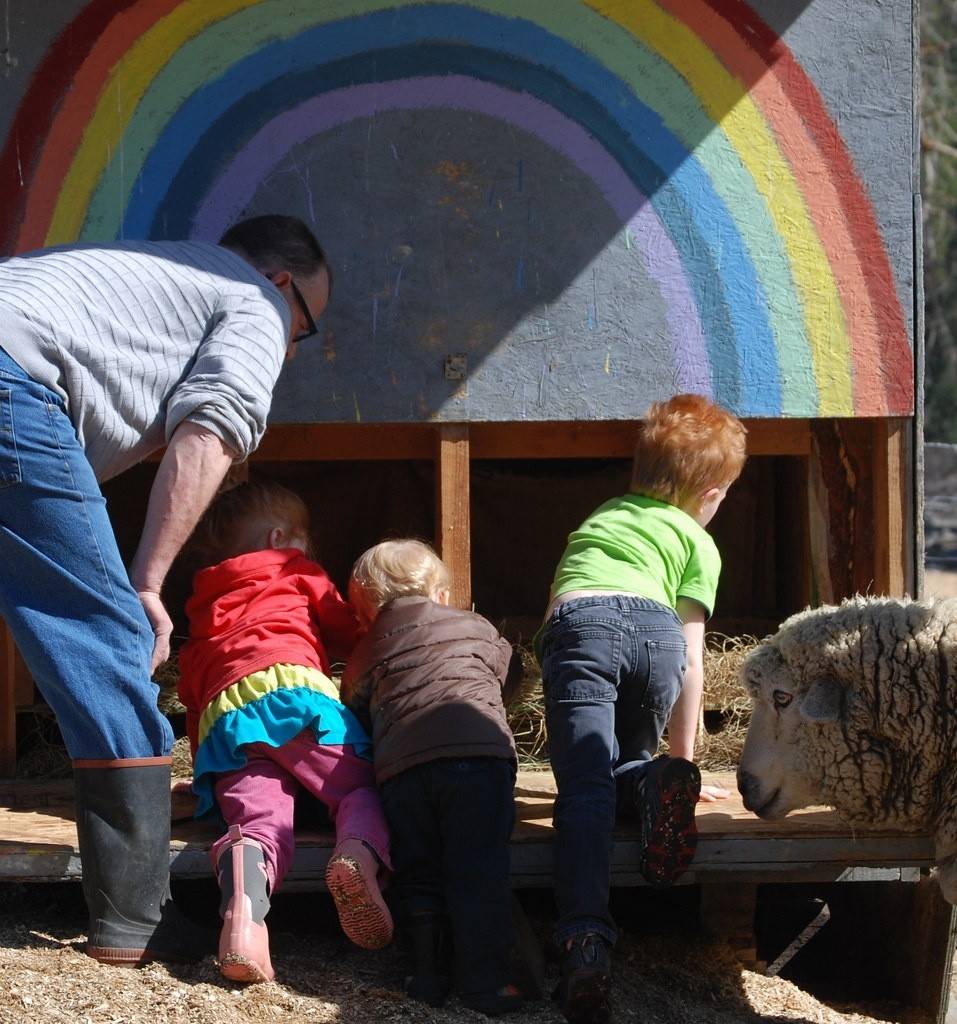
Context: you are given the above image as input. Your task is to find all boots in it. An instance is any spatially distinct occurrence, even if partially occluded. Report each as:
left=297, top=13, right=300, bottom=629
left=462, top=979, right=522, bottom=1015
left=216, top=825, right=273, bottom=985
left=72, top=755, right=219, bottom=967
left=402, top=913, right=445, bottom=1005
left=327, top=834, right=393, bottom=950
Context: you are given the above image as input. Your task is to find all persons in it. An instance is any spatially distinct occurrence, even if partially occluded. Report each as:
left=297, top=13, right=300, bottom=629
left=0, top=211, right=393, bottom=991
left=340, top=538, right=526, bottom=1017
left=532, top=391, right=750, bottom=1023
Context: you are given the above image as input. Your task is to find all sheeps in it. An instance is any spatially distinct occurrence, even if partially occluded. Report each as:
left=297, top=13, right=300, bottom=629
left=735, top=589, right=957, bottom=905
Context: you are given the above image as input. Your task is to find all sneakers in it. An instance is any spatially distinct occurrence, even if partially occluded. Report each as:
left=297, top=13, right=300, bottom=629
left=558, top=935, right=611, bottom=1020
left=632, top=755, right=702, bottom=888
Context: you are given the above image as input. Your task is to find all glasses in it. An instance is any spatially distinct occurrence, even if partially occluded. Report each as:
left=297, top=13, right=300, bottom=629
left=266, top=272, right=317, bottom=345
